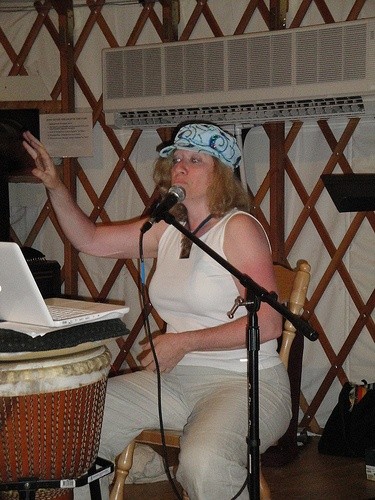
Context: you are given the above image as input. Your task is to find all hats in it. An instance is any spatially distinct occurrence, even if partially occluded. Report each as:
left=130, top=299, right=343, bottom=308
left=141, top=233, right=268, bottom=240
left=159, top=123, right=240, bottom=168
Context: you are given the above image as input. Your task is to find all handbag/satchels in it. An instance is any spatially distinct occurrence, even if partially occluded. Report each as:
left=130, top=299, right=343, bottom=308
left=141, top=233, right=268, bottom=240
left=318, top=382, right=375, bottom=465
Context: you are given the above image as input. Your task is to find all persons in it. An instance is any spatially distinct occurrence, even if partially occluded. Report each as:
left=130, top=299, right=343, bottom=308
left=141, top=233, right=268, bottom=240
left=23, top=122, right=291, bottom=500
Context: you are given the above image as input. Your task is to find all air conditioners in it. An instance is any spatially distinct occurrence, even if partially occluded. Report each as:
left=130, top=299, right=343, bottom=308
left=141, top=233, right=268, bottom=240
left=101, top=18, right=375, bottom=129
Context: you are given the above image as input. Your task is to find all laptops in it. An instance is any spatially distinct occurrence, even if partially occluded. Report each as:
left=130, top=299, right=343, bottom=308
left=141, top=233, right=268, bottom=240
left=0, top=241, right=130, bottom=328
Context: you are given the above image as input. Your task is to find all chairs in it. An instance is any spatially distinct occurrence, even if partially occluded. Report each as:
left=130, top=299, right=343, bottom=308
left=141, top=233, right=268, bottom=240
left=110, top=262, right=311, bottom=500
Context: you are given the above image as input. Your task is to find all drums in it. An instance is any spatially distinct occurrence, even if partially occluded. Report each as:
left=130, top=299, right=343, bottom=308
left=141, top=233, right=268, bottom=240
left=0, top=344, right=113, bottom=500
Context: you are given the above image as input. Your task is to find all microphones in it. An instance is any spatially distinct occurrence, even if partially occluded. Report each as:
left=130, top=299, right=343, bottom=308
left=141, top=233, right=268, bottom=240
left=140, top=186, right=186, bottom=232
